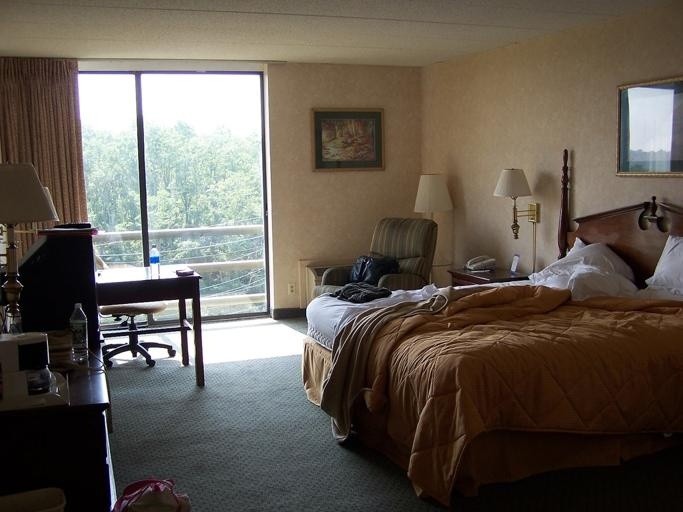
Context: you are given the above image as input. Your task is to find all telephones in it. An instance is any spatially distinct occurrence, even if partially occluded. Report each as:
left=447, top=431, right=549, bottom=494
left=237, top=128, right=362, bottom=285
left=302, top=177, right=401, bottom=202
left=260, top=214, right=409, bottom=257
left=465, top=255, right=497, bottom=270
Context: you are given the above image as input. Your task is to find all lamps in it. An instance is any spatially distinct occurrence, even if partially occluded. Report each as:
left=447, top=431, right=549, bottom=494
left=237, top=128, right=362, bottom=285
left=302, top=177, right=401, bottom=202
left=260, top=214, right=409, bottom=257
left=0, top=162, right=55, bottom=321
left=412, top=174, right=453, bottom=219
left=492, top=168, right=541, bottom=240
left=41, top=186, right=58, bottom=222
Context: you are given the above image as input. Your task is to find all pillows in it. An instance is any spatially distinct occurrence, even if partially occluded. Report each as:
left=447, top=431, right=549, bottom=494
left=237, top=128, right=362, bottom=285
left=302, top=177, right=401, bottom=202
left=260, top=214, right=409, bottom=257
left=565, top=237, right=585, bottom=257
left=526, top=243, right=633, bottom=287
left=644, top=234, right=681, bottom=294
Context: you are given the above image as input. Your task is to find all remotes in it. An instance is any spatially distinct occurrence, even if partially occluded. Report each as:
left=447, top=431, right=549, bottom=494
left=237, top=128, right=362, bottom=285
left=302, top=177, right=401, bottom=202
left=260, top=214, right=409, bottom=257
left=54, top=223, right=91, bottom=228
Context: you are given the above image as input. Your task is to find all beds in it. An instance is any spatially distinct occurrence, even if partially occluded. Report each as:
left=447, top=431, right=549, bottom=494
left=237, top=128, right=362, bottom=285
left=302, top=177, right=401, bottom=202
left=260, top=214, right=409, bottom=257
left=300, top=146, right=681, bottom=506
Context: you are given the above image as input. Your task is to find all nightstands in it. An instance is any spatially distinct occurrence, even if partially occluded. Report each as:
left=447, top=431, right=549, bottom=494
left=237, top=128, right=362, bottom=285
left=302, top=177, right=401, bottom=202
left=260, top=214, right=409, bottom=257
left=447, top=267, right=529, bottom=286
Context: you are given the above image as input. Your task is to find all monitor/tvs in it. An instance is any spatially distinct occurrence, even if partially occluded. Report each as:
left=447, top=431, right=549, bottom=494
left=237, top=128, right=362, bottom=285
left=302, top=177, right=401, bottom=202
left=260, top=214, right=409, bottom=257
left=0, top=235, right=100, bottom=345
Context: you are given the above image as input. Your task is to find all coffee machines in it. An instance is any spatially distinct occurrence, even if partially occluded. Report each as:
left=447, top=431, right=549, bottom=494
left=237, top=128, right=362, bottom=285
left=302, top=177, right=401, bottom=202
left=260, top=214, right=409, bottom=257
left=0, top=332, right=68, bottom=408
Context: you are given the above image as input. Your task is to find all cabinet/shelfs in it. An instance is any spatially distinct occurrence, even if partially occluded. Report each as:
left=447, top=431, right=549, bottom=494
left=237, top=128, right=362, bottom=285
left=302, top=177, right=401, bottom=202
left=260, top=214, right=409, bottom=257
left=0, top=324, right=111, bottom=511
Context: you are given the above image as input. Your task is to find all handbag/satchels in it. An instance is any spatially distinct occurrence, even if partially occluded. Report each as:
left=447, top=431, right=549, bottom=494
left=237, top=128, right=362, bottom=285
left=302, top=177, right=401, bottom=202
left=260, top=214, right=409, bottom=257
left=349, top=255, right=400, bottom=285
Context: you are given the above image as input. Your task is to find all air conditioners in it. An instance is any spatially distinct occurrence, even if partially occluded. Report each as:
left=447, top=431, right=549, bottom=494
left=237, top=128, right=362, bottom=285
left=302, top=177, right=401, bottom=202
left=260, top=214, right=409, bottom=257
left=305, top=264, right=353, bottom=307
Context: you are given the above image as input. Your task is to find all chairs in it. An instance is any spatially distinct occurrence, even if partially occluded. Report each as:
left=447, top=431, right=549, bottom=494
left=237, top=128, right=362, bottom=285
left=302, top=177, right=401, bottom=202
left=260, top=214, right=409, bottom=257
left=92, top=249, right=176, bottom=367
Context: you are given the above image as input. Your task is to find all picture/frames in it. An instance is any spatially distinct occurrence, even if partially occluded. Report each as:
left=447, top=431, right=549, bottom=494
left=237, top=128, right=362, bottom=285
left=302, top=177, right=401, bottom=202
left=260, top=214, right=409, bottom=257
left=310, top=106, right=384, bottom=172
left=615, top=75, right=682, bottom=177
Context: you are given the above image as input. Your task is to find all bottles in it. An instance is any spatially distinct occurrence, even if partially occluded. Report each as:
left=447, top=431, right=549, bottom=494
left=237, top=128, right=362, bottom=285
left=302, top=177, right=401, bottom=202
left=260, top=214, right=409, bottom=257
left=69, top=302, right=87, bottom=364
left=148, top=244, right=159, bottom=275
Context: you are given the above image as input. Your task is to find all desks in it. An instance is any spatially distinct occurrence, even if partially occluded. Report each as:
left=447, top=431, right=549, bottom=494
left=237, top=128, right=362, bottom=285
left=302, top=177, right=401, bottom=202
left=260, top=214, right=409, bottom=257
left=92, top=265, right=203, bottom=385
left=426, top=261, right=451, bottom=286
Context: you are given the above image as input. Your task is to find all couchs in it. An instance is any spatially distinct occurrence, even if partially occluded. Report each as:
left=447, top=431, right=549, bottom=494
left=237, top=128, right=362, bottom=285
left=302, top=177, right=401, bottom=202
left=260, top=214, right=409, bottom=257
left=311, top=216, right=437, bottom=296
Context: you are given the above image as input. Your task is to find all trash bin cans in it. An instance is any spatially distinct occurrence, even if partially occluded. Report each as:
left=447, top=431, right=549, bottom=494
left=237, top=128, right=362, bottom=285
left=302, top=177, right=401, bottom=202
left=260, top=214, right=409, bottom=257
left=0, top=487, right=66, bottom=512
